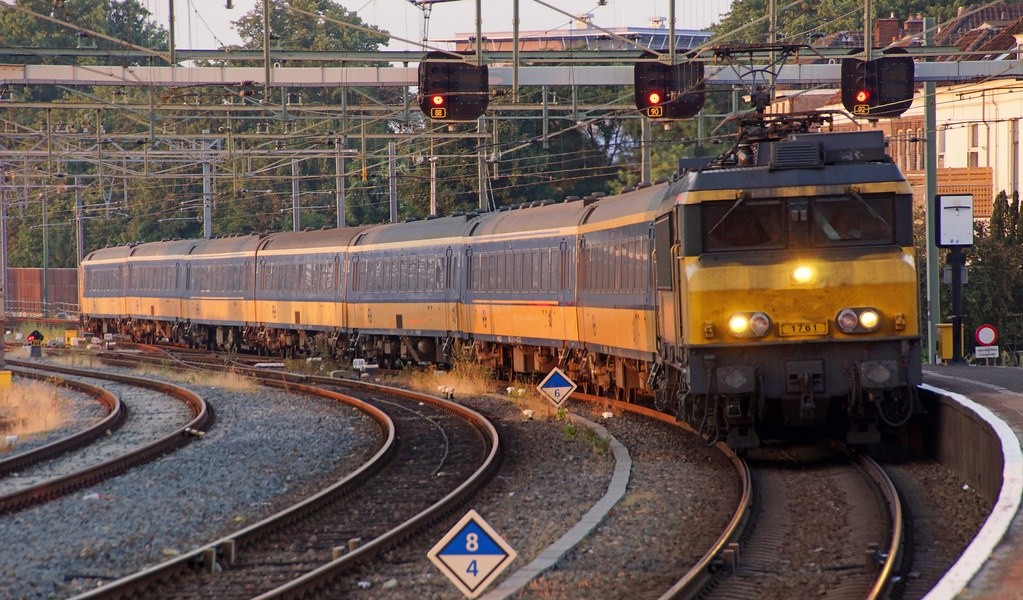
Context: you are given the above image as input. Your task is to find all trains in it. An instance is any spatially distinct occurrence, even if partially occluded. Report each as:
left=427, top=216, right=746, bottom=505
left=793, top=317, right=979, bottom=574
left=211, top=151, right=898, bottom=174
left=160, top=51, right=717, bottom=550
left=0, top=127, right=927, bottom=450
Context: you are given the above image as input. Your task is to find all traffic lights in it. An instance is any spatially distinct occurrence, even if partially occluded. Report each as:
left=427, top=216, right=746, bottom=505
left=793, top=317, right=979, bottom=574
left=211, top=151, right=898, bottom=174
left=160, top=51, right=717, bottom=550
left=646, top=62, right=666, bottom=108
left=429, top=62, right=447, bottom=107
left=856, top=59, right=870, bottom=103
